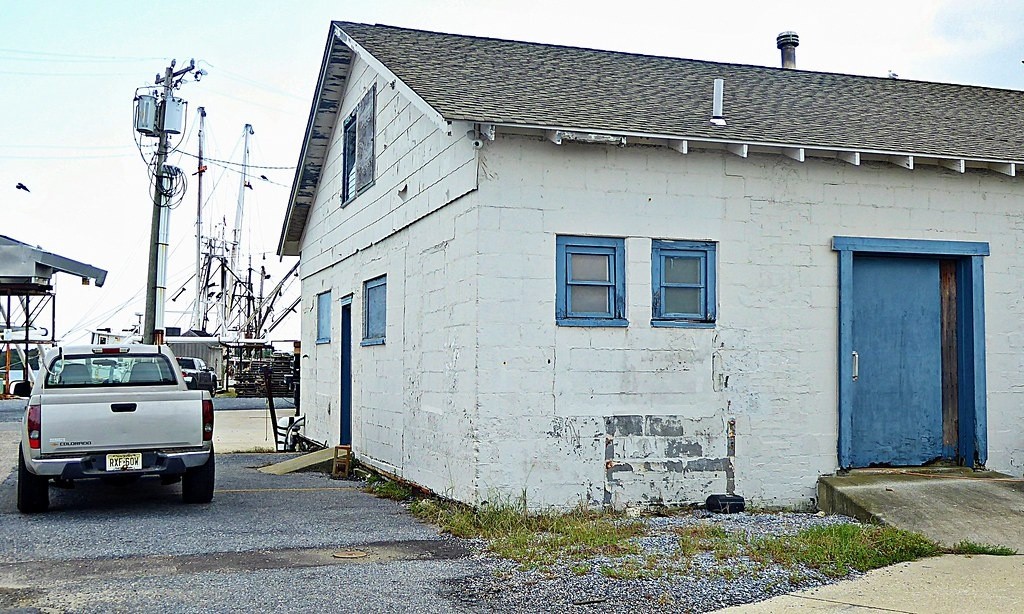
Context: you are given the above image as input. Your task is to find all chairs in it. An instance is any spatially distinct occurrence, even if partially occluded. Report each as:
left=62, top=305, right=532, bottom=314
left=129, top=362, right=161, bottom=381
left=60, top=364, right=92, bottom=385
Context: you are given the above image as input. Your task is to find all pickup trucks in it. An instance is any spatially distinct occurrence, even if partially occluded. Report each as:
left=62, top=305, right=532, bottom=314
left=156, top=357, right=217, bottom=397
left=18, top=344, right=215, bottom=516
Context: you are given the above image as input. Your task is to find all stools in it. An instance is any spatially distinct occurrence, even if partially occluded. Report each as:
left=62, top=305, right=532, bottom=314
left=333, top=445, right=351, bottom=478
